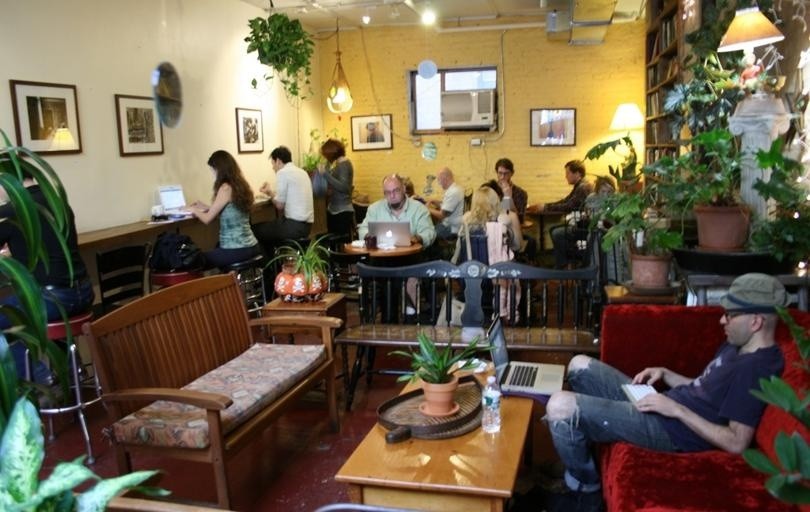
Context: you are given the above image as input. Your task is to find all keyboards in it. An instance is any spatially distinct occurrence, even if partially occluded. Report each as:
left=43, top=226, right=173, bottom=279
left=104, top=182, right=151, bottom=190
left=621, top=383, right=658, bottom=412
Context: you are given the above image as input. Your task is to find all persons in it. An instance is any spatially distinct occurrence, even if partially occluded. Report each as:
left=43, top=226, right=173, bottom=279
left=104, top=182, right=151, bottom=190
left=542, top=273, right=790, bottom=512
left=365, top=123, right=384, bottom=143
left=316, top=140, right=355, bottom=243
left=0, top=149, right=95, bottom=398
left=357, top=173, right=437, bottom=322
left=178, top=150, right=261, bottom=271
left=252, top=146, right=314, bottom=252
left=401, top=159, right=618, bottom=321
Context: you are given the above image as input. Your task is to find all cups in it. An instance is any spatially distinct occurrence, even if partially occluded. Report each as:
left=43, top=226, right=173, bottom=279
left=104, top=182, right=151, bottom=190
left=364, top=233, right=377, bottom=251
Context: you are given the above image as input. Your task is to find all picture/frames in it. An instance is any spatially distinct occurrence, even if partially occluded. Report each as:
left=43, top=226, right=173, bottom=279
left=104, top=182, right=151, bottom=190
left=115, top=94, right=164, bottom=157
left=351, top=113, right=393, bottom=150
left=8, top=80, right=83, bottom=157
left=235, top=108, right=265, bottom=153
left=530, top=108, right=576, bottom=147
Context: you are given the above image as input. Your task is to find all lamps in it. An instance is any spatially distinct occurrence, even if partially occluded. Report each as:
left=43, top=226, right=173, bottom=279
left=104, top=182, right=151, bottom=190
left=715, top=6, right=787, bottom=97
left=609, top=103, right=644, bottom=181
left=325, top=16, right=355, bottom=114
left=417, top=0, right=438, bottom=80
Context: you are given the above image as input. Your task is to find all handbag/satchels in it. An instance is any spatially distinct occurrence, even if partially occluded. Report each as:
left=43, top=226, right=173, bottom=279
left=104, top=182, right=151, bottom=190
left=434, top=296, right=466, bottom=325
left=152, top=230, right=206, bottom=272
left=312, top=164, right=330, bottom=193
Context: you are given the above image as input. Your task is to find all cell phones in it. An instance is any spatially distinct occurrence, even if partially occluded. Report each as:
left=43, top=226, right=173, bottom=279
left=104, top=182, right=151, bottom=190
left=171, top=215, right=185, bottom=218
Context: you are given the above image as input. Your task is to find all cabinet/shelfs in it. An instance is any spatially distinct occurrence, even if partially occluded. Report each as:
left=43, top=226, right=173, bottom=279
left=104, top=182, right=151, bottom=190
left=645, top=1, right=684, bottom=196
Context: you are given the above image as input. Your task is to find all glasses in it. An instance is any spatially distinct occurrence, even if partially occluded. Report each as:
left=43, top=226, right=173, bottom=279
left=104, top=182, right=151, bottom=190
left=497, top=172, right=513, bottom=176
left=725, top=310, right=759, bottom=321
left=384, top=186, right=400, bottom=195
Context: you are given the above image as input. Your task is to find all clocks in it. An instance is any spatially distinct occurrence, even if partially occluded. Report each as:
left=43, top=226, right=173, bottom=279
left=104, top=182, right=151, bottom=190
left=151, top=60, right=184, bottom=129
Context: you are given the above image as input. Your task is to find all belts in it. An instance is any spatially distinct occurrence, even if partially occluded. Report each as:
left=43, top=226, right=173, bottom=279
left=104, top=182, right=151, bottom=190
left=42, top=274, right=91, bottom=289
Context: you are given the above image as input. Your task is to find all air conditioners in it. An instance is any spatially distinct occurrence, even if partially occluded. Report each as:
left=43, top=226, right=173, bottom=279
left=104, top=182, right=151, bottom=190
left=440, top=88, right=497, bottom=129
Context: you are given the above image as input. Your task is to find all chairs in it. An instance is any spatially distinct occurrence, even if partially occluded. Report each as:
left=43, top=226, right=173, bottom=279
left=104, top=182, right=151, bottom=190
left=324, top=197, right=565, bottom=323
left=89, top=242, right=148, bottom=320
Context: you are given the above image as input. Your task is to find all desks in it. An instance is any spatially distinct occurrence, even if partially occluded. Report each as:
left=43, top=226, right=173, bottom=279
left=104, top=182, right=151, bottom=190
left=0, top=192, right=276, bottom=260
left=685, top=266, right=809, bottom=312
left=603, top=282, right=684, bottom=305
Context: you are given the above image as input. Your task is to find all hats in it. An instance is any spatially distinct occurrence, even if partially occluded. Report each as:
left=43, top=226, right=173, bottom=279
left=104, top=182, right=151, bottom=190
left=719, top=273, right=793, bottom=309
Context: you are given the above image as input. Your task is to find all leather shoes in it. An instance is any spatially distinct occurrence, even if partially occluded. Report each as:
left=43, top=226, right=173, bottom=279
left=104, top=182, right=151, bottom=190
left=539, top=469, right=605, bottom=511
left=36, top=364, right=90, bottom=391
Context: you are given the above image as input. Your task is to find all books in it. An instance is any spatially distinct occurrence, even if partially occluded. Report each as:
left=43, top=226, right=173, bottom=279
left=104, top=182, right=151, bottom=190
left=645, top=0, right=682, bottom=212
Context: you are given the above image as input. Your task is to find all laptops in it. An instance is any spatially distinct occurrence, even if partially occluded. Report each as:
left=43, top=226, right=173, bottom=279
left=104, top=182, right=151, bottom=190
left=158, top=184, right=208, bottom=215
left=487, top=313, right=566, bottom=396
left=368, top=222, right=413, bottom=246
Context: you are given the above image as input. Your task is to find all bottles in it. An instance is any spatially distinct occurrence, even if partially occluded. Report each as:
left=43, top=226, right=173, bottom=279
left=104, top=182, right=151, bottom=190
left=479, top=375, right=503, bottom=436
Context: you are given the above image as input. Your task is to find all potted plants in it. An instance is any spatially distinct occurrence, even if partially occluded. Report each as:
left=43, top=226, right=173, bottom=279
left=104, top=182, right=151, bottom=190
left=578, top=135, right=710, bottom=296
left=385, top=326, right=499, bottom=416
left=262, top=229, right=338, bottom=303
left=653, top=127, right=764, bottom=255
left=242, top=0, right=316, bottom=111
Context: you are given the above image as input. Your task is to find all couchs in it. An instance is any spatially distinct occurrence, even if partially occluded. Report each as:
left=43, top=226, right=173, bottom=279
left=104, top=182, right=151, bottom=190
left=594, top=304, right=810, bottom=511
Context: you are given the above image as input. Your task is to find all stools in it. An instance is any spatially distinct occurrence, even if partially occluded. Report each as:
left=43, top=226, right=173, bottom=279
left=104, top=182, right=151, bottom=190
left=23, top=309, right=112, bottom=465
left=216, top=254, right=265, bottom=318
left=149, top=269, right=204, bottom=286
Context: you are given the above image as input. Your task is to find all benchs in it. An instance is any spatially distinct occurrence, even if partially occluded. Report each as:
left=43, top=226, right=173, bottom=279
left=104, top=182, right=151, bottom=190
left=81, top=268, right=343, bottom=510
left=335, top=258, right=601, bottom=412
